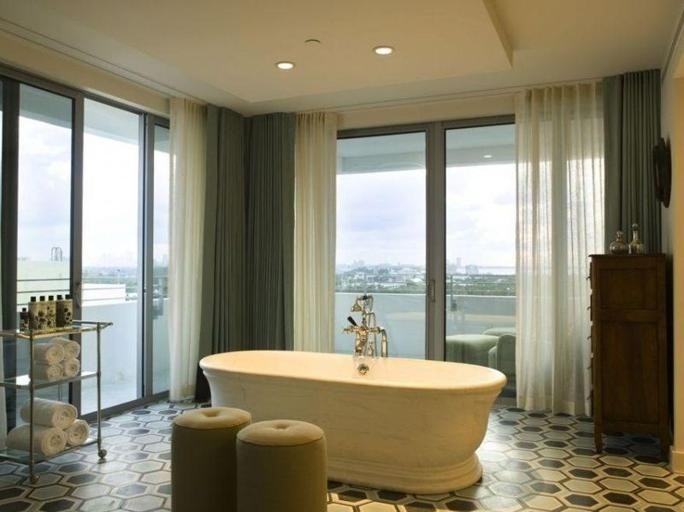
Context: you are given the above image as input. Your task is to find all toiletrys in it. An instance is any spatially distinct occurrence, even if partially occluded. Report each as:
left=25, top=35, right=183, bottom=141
left=20, top=294, right=73, bottom=332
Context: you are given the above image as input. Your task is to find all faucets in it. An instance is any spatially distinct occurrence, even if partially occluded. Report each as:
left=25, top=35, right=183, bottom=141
left=356, top=340, right=366, bottom=353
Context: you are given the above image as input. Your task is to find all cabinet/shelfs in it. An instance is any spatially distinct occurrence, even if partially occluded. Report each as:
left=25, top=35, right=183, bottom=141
left=587, top=252, right=669, bottom=456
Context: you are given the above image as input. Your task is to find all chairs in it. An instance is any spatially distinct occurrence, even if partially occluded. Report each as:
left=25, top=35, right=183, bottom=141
left=483, top=330, right=516, bottom=396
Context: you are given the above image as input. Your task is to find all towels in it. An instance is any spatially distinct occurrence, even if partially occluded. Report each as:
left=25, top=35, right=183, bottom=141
left=5, top=398, right=90, bottom=457
left=29, top=337, right=80, bottom=382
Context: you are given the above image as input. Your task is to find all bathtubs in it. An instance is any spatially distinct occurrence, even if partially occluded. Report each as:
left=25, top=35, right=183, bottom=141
left=199, top=349, right=507, bottom=495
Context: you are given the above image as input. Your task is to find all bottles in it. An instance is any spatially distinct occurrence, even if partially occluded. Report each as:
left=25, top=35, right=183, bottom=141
left=20, top=295, right=73, bottom=334
left=609, top=224, right=646, bottom=255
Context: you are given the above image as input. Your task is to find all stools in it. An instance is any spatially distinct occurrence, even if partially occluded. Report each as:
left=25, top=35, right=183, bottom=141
left=236, top=418, right=327, bottom=512
left=171, top=408, right=251, bottom=511
left=445, top=335, right=498, bottom=366
